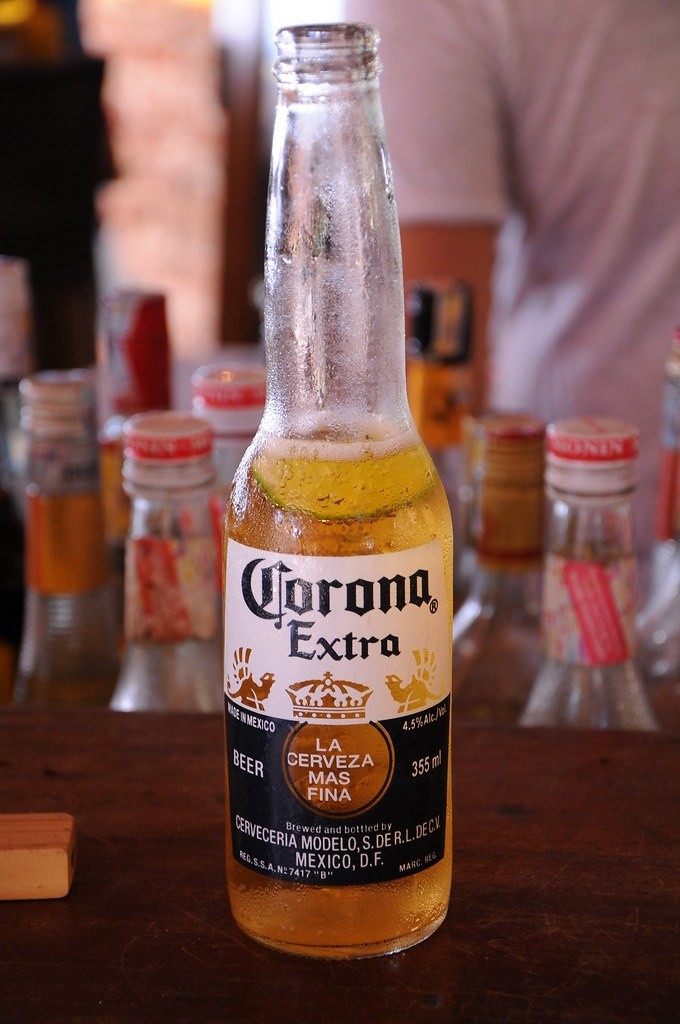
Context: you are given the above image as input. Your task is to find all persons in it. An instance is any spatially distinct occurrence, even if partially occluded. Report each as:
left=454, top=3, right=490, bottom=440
left=344, top=0, right=680, bottom=564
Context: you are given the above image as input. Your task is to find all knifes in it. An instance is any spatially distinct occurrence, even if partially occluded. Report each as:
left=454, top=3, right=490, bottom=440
left=407, top=279, right=473, bottom=365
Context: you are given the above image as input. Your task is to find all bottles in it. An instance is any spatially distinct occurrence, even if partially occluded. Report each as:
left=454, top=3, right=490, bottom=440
left=15, top=367, right=268, bottom=712
left=223, top=25, right=451, bottom=958
left=452, top=328, right=680, bottom=733
